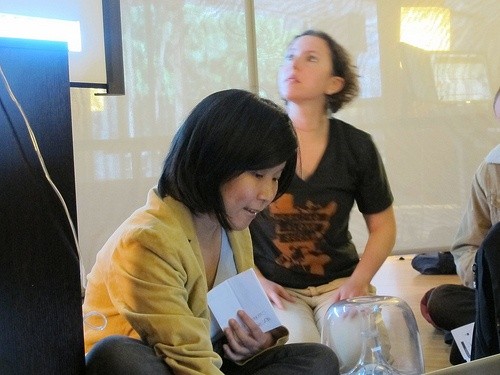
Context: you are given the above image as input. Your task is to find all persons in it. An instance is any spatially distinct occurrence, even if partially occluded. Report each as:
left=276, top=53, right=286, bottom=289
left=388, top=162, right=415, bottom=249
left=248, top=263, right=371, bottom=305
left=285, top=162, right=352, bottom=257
left=248, top=30, right=397, bottom=375
left=428, top=88, right=500, bottom=365
left=82, top=89, right=341, bottom=375
